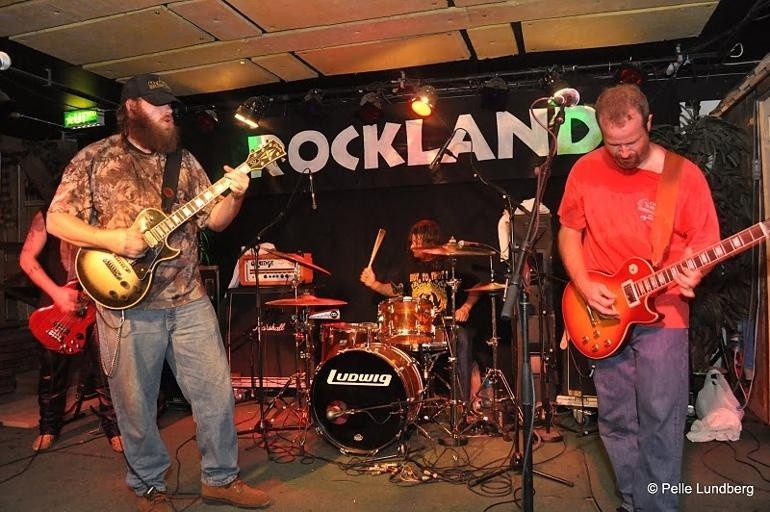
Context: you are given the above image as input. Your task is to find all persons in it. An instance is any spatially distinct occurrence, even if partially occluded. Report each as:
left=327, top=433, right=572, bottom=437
left=359, top=219, right=494, bottom=420
left=18, top=204, right=123, bottom=454
left=45, top=75, right=274, bottom=512
left=557, top=83, right=720, bottom=512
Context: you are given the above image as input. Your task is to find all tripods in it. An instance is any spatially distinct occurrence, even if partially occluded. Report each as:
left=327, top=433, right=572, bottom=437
left=235, top=337, right=276, bottom=409
left=263, top=276, right=312, bottom=420
left=468, top=148, right=576, bottom=488
left=506, top=307, right=583, bottom=437
left=237, top=186, right=312, bottom=460
left=452, top=301, right=524, bottom=432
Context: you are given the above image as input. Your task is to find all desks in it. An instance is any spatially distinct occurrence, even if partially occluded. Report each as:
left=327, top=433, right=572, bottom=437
left=223, top=284, right=318, bottom=424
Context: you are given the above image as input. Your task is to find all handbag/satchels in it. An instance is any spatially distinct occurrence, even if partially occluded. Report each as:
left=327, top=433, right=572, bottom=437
left=695, top=370, right=744, bottom=424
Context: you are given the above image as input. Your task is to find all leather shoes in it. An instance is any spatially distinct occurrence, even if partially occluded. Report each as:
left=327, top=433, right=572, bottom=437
left=137, top=492, right=176, bottom=512
left=111, top=434, right=124, bottom=452
left=201, top=476, right=270, bottom=508
left=32, top=434, right=57, bottom=450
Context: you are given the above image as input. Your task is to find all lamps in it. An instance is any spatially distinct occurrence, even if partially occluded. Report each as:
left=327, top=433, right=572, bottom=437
left=232, top=95, right=265, bottom=130
left=411, top=84, right=439, bottom=114
left=548, top=78, right=583, bottom=108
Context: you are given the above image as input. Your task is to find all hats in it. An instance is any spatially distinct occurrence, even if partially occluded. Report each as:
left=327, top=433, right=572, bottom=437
left=120, top=74, right=182, bottom=106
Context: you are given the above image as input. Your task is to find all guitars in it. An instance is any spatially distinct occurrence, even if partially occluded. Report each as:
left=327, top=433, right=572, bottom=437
left=74, top=139, right=287, bottom=310
left=561, top=218, right=770, bottom=360
left=29, top=276, right=97, bottom=356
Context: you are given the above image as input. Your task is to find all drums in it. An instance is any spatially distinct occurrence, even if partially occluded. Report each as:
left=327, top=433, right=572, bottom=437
left=321, top=323, right=380, bottom=357
left=422, top=327, right=447, bottom=347
left=309, top=343, right=426, bottom=456
left=377, top=297, right=434, bottom=346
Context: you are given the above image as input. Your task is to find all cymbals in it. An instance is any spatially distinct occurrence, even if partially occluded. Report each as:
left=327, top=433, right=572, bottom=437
left=465, top=282, right=506, bottom=292
left=415, top=248, right=490, bottom=257
left=261, top=247, right=330, bottom=276
left=264, top=296, right=346, bottom=306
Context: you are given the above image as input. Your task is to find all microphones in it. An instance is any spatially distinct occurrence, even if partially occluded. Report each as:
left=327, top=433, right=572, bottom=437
left=552, top=88, right=581, bottom=108
left=326, top=411, right=344, bottom=420
left=458, top=240, right=480, bottom=249
left=428, top=132, right=456, bottom=170
left=309, top=174, right=318, bottom=210
